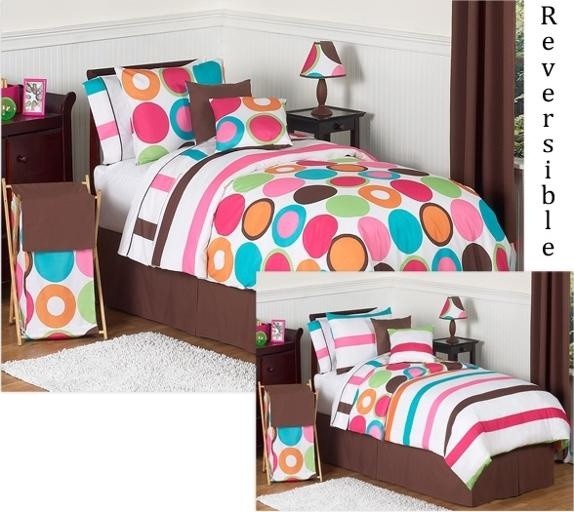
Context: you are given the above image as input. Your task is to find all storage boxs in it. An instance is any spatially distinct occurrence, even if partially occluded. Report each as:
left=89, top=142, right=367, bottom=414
left=0, top=84, right=21, bottom=114
left=256, top=325, right=270, bottom=342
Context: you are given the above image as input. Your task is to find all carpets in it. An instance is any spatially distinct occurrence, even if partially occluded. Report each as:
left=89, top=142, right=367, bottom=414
left=256, top=475, right=455, bottom=511
left=1, top=331, right=256, bottom=392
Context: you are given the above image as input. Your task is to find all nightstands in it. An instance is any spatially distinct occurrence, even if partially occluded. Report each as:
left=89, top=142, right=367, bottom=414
left=433, top=336, right=478, bottom=367
left=286, top=105, right=366, bottom=151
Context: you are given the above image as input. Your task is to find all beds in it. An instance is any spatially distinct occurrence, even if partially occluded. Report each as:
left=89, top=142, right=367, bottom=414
left=87, top=61, right=517, bottom=356
left=307, top=307, right=571, bottom=508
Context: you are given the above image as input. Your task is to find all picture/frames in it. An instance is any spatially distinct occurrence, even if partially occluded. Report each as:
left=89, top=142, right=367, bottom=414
left=22, top=77, right=47, bottom=117
left=270, top=320, right=285, bottom=345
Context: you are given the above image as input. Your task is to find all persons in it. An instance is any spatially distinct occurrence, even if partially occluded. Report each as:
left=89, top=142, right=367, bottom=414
left=24, top=83, right=43, bottom=112
left=272, top=321, right=283, bottom=339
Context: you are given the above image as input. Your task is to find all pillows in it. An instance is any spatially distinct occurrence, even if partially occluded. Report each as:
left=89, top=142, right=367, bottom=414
left=82, top=75, right=136, bottom=167
left=307, top=305, right=436, bottom=375
left=183, top=79, right=254, bottom=147
left=208, top=97, right=292, bottom=153
left=113, top=57, right=228, bottom=166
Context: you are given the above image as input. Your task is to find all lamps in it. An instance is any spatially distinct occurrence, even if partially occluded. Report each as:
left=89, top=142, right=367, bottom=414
left=299, top=41, right=347, bottom=118
left=438, top=296, right=468, bottom=344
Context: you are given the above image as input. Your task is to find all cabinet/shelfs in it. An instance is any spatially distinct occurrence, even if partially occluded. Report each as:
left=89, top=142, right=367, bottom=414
left=0, top=90, right=73, bottom=302
left=256, top=327, right=305, bottom=459
